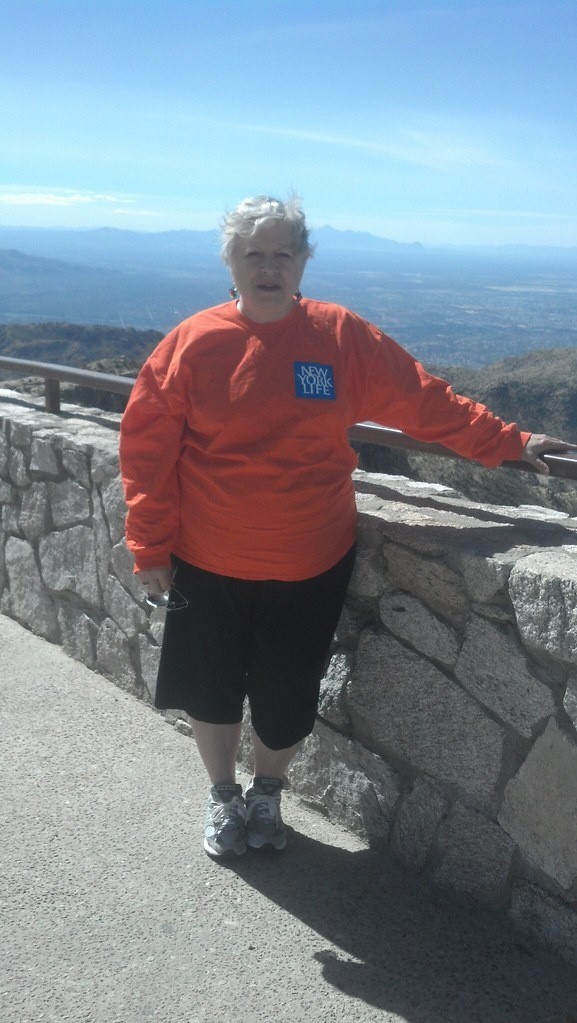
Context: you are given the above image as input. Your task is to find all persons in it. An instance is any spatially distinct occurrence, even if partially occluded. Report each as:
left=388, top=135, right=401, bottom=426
left=118, top=195, right=577, bottom=859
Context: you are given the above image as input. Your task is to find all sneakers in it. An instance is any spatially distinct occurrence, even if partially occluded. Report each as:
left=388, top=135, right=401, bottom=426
left=244, top=775, right=288, bottom=851
left=204, top=783, right=246, bottom=857
